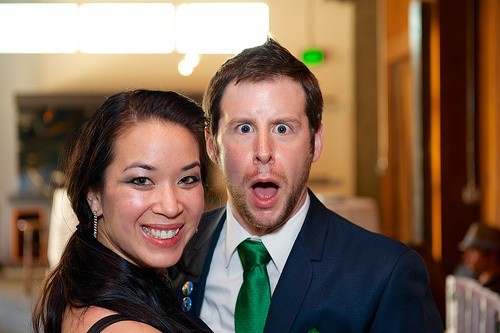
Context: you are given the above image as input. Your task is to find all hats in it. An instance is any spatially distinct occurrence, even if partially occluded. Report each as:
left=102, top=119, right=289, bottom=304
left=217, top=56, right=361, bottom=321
left=458, top=223, right=500, bottom=252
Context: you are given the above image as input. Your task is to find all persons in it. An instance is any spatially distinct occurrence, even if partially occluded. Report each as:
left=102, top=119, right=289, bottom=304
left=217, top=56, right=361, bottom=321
left=31, top=89, right=214, bottom=333
left=457, top=222, right=500, bottom=295
left=161, top=34, right=446, bottom=333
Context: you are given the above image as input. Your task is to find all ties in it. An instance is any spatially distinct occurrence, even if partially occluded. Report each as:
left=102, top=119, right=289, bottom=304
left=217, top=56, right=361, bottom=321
left=234, top=238, right=272, bottom=333
